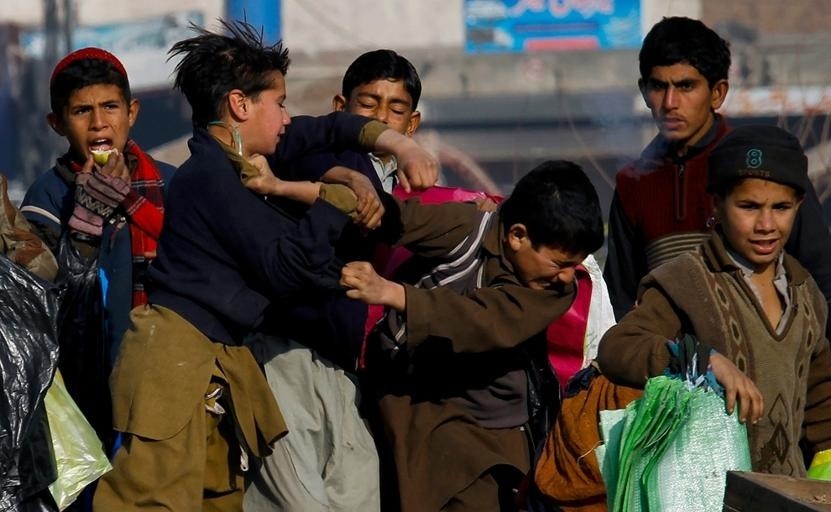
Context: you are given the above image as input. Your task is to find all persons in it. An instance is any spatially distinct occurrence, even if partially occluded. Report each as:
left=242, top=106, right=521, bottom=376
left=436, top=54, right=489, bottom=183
left=91, top=17, right=440, bottom=512
left=21, top=46, right=177, bottom=452
left=272, top=46, right=497, bottom=369
left=601, top=14, right=830, bottom=333
left=594, top=122, right=831, bottom=481
left=239, top=151, right=607, bottom=512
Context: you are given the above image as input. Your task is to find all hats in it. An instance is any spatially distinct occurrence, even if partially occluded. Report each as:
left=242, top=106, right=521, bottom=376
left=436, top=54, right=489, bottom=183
left=708, top=123, right=808, bottom=195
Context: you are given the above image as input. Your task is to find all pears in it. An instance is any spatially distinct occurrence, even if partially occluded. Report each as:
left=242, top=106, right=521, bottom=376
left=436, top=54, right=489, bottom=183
left=92, top=150, right=112, bottom=165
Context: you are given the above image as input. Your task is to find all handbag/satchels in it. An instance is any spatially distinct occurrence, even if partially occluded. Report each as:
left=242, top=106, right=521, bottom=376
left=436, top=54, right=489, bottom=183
left=522, top=366, right=562, bottom=468
left=535, top=334, right=750, bottom=512
